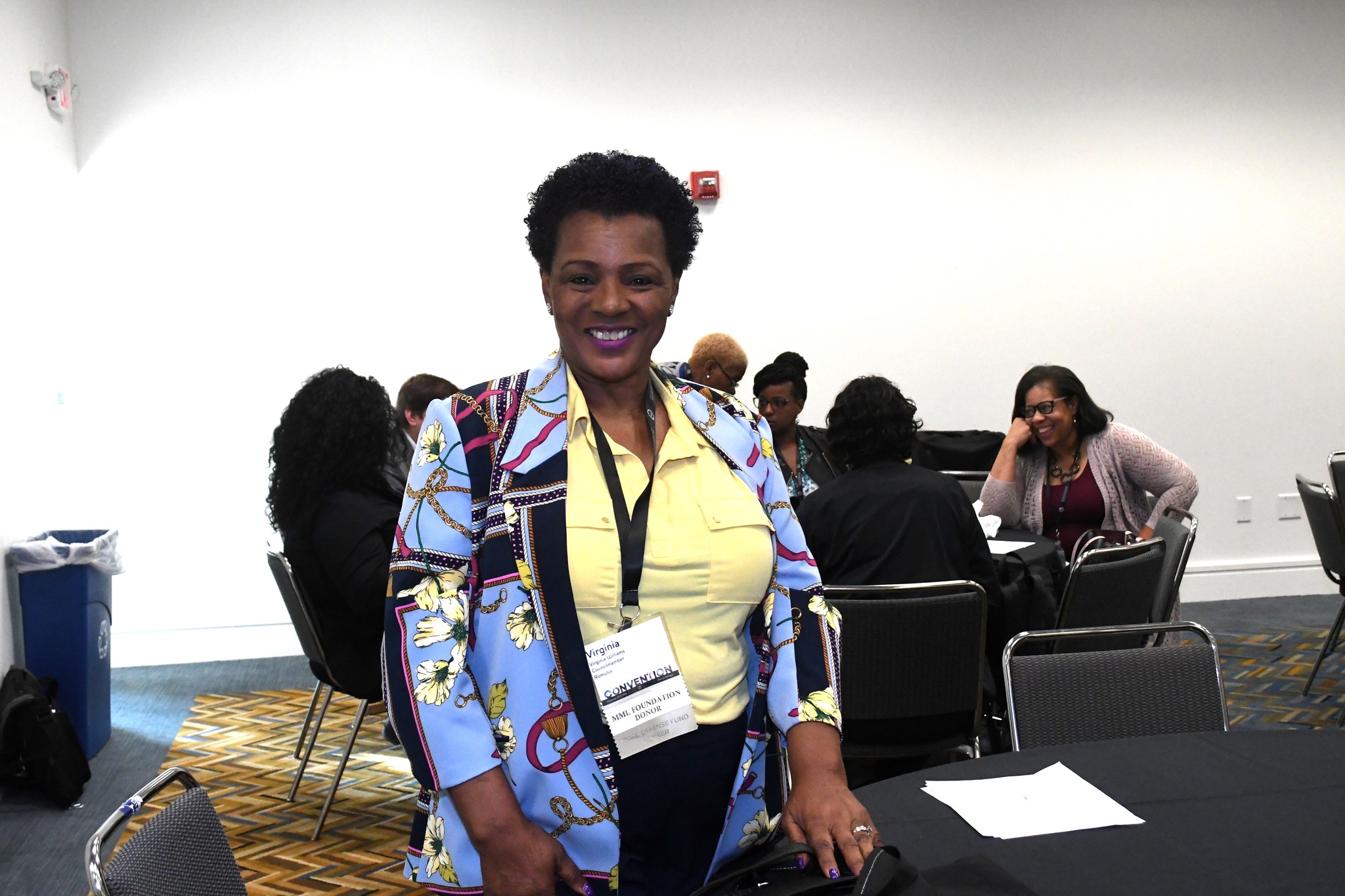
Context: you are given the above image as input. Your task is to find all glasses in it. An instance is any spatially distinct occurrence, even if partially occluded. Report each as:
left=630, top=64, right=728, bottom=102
left=1020, top=396, right=1070, bottom=418
left=753, top=397, right=802, bottom=410
left=714, top=360, right=739, bottom=390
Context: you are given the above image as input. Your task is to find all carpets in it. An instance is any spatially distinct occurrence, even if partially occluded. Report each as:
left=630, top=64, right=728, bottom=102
left=87, top=629, right=1345, bottom=896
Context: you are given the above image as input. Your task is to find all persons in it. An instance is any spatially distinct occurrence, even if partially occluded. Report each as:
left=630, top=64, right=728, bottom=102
left=263, top=366, right=408, bottom=748
left=380, top=150, right=883, bottom=896
left=795, top=372, right=1010, bottom=753
left=978, top=365, right=1205, bottom=601
left=751, top=351, right=839, bottom=513
left=383, top=375, right=462, bottom=497
left=653, top=333, right=747, bottom=397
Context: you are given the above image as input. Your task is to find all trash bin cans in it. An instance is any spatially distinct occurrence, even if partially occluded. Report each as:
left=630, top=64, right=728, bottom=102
left=11, top=529, right=124, bottom=759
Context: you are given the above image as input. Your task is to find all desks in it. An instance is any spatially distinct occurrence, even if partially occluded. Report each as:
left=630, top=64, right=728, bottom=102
left=984, top=529, right=1065, bottom=611
left=705, top=728, right=1345, bottom=896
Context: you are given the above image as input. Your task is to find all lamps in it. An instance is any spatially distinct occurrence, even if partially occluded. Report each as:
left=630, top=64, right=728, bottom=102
left=30, top=65, right=80, bottom=115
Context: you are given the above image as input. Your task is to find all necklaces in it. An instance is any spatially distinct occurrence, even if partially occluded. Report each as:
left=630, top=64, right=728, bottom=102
left=1046, top=443, right=1082, bottom=481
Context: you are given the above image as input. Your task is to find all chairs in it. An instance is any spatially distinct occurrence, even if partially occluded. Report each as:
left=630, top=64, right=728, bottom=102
left=1048, top=536, right=1166, bottom=656
left=266, top=542, right=382, bottom=842
left=938, top=470, right=989, bottom=505
left=781, top=581, right=988, bottom=803
left=85, top=766, right=249, bottom=896
left=1294, top=451, right=1345, bottom=697
left=1145, top=504, right=1197, bottom=648
left=1001, top=621, right=1230, bottom=751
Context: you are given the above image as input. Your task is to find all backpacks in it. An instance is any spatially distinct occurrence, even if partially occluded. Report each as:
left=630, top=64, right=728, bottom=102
left=985, top=551, right=1058, bottom=705
left=0, top=667, right=95, bottom=811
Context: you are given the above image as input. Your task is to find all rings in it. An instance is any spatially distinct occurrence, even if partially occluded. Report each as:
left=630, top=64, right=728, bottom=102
left=852, top=824, right=873, bottom=838
